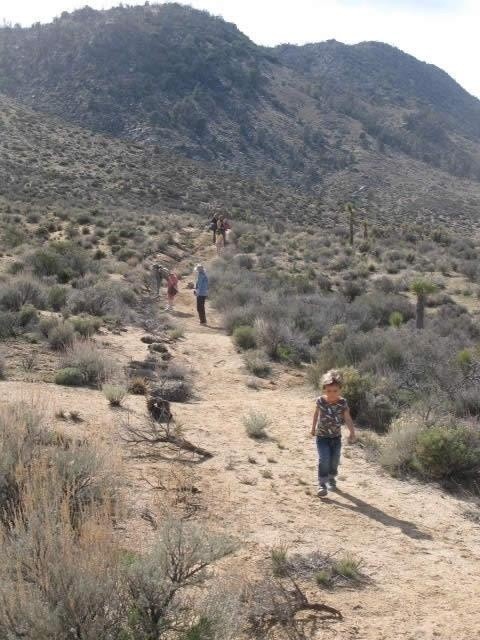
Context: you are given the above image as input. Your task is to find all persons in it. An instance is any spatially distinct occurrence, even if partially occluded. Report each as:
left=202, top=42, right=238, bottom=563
left=309, top=368, right=356, bottom=495
left=208, top=212, right=231, bottom=253
left=192, top=264, right=209, bottom=326
left=160, top=268, right=178, bottom=311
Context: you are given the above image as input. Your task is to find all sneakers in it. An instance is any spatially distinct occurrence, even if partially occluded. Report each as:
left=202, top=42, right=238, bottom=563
left=317, top=484, right=327, bottom=496
left=326, top=479, right=337, bottom=492
